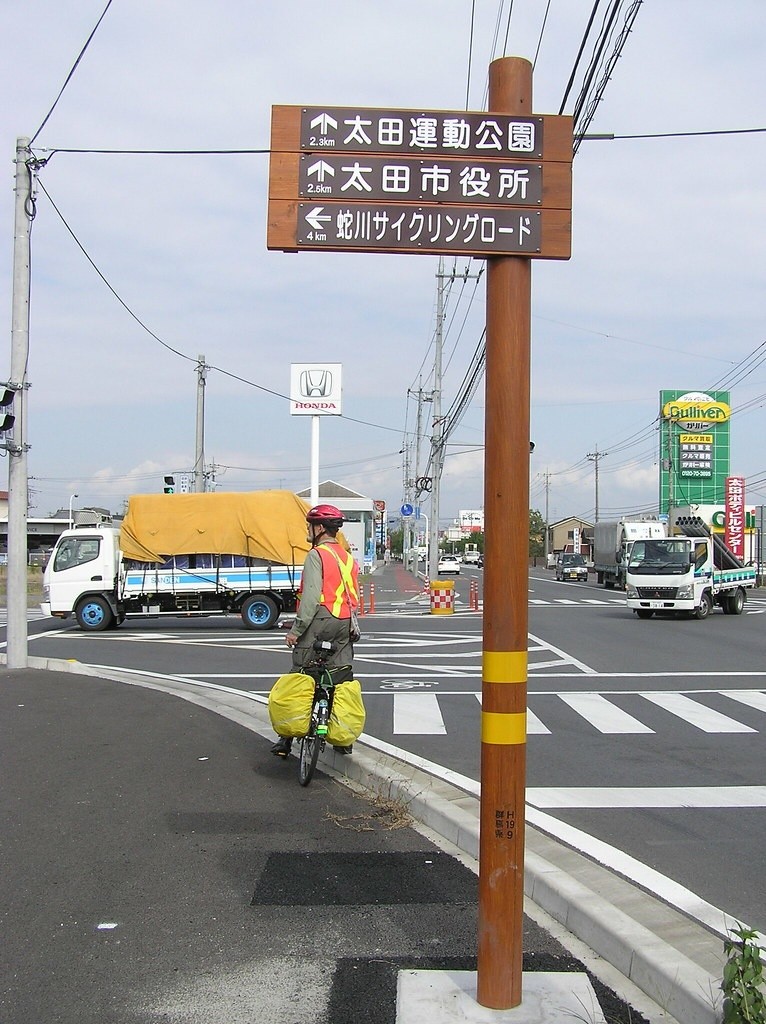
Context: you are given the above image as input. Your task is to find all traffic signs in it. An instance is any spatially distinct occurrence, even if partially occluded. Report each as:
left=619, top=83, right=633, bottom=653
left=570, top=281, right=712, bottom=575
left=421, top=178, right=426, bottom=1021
left=297, top=107, right=543, bottom=161
left=296, top=202, right=543, bottom=253
left=297, top=156, right=544, bottom=207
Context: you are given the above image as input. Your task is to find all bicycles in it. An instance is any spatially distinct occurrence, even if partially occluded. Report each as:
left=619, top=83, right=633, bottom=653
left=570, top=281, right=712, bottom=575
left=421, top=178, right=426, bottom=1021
left=276, top=619, right=337, bottom=787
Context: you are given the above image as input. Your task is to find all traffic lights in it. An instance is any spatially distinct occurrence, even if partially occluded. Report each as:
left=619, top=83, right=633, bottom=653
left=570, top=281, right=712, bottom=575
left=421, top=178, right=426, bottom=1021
left=530, top=442, right=536, bottom=455
left=164, top=476, right=174, bottom=494
left=0, top=386, right=16, bottom=432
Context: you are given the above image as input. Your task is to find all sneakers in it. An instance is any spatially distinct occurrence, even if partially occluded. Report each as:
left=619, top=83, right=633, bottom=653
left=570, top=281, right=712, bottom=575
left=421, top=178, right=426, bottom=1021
left=272, top=744, right=290, bottom=756
left=333, top=744, right=352, bottom=754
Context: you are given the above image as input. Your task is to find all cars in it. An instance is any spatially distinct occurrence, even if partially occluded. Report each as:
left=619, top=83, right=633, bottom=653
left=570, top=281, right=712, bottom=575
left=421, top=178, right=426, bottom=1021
left=437, top=556, right=461, bottom=575
left=453, top=551, right=484, bottom=568
left=410, top=554, right=426, bottom=563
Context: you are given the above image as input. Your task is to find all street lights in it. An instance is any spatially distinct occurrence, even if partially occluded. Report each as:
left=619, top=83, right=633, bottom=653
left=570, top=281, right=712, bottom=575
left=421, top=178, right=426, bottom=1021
left=68, top=492, right=80, bottom=529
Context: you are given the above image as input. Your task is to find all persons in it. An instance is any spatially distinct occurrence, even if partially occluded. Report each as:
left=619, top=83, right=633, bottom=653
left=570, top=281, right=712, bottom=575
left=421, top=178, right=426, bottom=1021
left=270, top=506, right=361, bottom=756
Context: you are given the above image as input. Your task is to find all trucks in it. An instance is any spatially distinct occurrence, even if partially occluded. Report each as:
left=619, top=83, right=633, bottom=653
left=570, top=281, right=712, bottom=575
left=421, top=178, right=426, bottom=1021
left=594, top=519, right=669, bottom=591
left=615, top=535, right=757, bottom=620
left=38, top=528, right=304, bottom=632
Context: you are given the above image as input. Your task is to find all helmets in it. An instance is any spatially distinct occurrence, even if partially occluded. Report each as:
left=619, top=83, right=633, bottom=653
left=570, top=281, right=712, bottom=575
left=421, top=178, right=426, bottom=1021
left=306, top=505, right=345, bottom=520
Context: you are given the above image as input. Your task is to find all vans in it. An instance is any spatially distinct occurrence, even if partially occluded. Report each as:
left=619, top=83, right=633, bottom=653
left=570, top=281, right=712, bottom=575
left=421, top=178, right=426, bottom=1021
left=556, top=552, right=588, bottom=582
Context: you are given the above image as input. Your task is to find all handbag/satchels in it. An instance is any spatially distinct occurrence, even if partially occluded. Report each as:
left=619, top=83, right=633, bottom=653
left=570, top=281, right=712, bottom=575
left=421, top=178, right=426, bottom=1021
left=350, top=609, right=360, bottom=642
left=269, top=667, right=315, bottom=737
left=325, top=680, right=366, bottom=746
